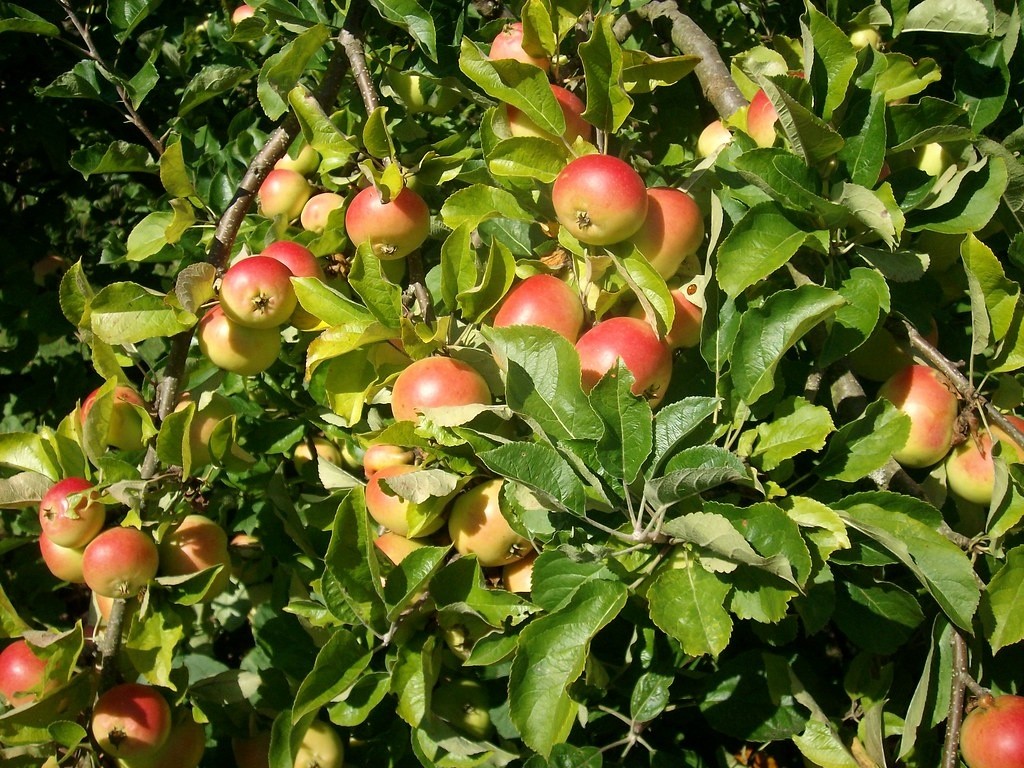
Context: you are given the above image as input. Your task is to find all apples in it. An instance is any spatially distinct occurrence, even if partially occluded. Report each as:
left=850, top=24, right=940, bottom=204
left=0, top=5, right=1024, bottom=768
